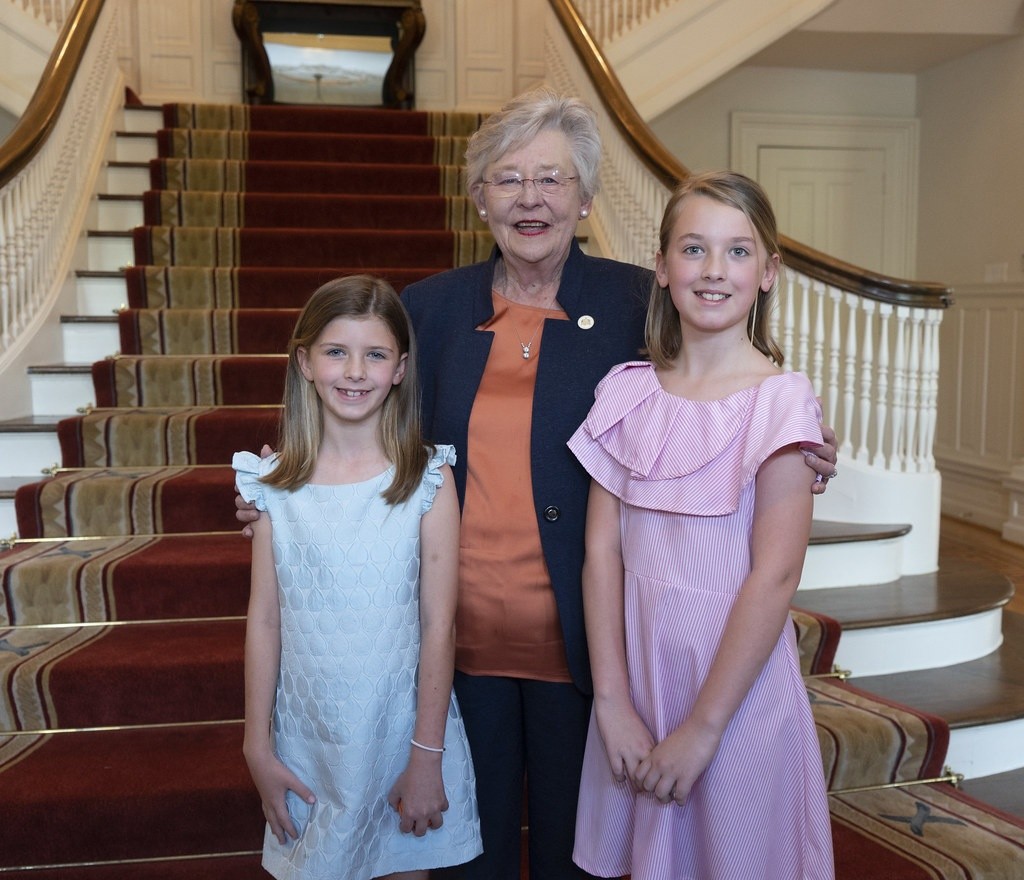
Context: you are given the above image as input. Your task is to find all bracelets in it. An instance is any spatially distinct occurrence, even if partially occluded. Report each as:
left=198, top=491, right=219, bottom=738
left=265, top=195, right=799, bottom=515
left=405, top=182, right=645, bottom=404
left=410, top=738, right=446, bottom=752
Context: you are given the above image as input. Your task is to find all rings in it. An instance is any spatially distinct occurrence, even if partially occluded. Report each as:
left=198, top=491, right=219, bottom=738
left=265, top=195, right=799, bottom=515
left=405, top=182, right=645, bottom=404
left=827, top=463, right=838, bottom=478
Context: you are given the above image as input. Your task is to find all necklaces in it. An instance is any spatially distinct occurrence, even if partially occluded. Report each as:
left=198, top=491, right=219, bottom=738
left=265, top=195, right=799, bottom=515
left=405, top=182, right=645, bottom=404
left=501, top=262, right=556, bottom=360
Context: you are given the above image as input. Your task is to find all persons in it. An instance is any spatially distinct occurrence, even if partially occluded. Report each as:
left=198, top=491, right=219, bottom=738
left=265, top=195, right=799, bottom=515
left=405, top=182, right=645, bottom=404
left=231, top=276, right=485, bottom=880
left=566, top=172, right=835, bottom=880
left=234, top=94, right=838, bottom=880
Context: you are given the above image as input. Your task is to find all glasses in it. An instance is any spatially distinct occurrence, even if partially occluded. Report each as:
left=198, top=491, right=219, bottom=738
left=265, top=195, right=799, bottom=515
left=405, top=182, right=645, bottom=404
left=479, top=170, right=578, bottom=198
